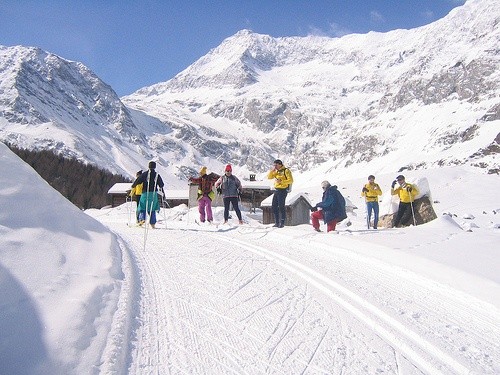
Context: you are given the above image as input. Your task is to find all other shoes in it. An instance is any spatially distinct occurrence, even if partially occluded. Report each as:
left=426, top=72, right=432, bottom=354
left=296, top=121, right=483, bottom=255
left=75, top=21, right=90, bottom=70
left=222, top=221, right=228, bottom=225
left=138, top=220, right=145, bottom=226
left=315, top=228, right=322, bottom=232
left=239, top=220, right=243, bottom=225
left=151, top=224, right=155, bottom=229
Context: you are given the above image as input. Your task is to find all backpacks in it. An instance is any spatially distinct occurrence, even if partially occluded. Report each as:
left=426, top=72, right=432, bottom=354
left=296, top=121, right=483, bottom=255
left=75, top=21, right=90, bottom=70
left=284, top=168, right=292, bottom=193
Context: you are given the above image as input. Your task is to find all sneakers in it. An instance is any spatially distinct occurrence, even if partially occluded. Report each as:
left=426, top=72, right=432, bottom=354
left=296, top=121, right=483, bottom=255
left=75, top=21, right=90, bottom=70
left=272, top=223, right=280, bottom=227
left=278, top=223, right=284, bottom=228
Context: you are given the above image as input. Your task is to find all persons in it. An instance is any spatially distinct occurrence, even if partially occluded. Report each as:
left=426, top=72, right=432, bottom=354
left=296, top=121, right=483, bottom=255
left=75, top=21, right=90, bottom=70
left=390, top=175, right=419, bottom=229
left=361, top=175, right=382, bottom=228
left=129, top=162, right=164, bottom=229
left=189, top=166, right=217, bottom=223
left=215, top=164, right=242, bottom=224
left=311, top=181, right=347, bottom=233
left=267, top=160, right=293, bottom=228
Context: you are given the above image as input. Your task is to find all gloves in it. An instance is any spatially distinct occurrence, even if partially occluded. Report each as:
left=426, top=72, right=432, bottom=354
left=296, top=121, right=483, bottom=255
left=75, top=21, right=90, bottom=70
left=126, top=190, right=130, bottom=196
left=217, top=188, right=222, bottom=193
left=406, top=186, right=412, bottom=193
left=238, top=192, right=242, bottom=198
left=391, top=180, right=397, bottom=188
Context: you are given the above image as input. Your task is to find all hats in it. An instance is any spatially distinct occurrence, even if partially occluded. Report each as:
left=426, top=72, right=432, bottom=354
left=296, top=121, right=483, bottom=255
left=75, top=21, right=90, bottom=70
left=225, top=165, right=232, bottom=172
left=273, top=160, right=282, bottom=166
left=396, top=175, right=405, bottom=180
left=200, top=166, right=207, bottom=175
left=136, top=170, right=142, bottom=174
